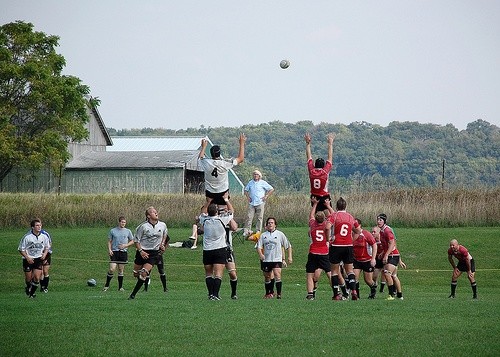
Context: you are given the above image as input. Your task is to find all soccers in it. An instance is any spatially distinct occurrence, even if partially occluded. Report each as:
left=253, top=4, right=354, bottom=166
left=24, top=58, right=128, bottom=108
left=279, top=59, right=290, bottom=69
left=87, top=279, right=96, bottom=286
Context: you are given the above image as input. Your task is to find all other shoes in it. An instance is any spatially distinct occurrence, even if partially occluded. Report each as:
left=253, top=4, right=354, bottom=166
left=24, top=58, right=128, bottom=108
left=385, top=295, right=396, bottom=300
left=43, top=286, right=48, bottom=294
left=306, top=294, right=314, bottom=301
left=393, top=288, right=397, bottom=295
left=333, top=288, right=360, bottom=301
left=277, top=294, right=281, bottom=299
left=370, top=283, right=376, bottom=294
left=396, top=293, right=403, bottom=300
left=40, top=285, right=43, bottom=292
left=264, top=294, right=269, bottom=299
left=164, top=288, right=169, bottom=292
left=128, top=295, right=134, bottom=300
left=367, top=295, right=375, bottom=300
left=29, top=294, right=36, bottom=298
left=269, top=291, right=275, bottom=298
left=380, top=281, right=385, bottom=293
left=231, top=294, right=238, bottom=300
left=118, top=287, right=124, bottom=291
left=209, top=295, right=222, bottom=301
left=189, top=236, right=196, bottom=240
left=25, top=284, right=31, bottom=295
left=447, top=295, right=456, bottom=298
left=191, top=246, right=197, bottom=249
left=103, top=286, right=109, bottom=292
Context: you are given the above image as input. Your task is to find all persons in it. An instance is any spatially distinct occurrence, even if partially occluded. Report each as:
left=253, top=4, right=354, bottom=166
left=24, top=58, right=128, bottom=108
left=352, top=218, right=378, bottom=299
left=128, top=206, right=168, bottom=300
left=325, top=196, right=359, bottom=301
left=306, top=195, right=342, bottom=300
left=247, top=221, right=287, bottom=296
left=200, top=191, right=234, bottom=301
left=257, top=217, right=293, bottom=299
left=447, top=239, right=476, bottom=300
left=198, top=133, right=246, bottom=215
left=241, top=169, right=275, bottom=240
left=189, top=206, right=209, bottom=250
left=212, top=203, right=240, bottom=300
left=103, top=216, right=134, bottom=291
left=141, top=235, right=171, bottom=292
left=370, top=224, right=406, bottom=295
left=304, top=130, right=334, bottom=217
left=17, top=219, right=51, bottom=299
left=19, top=217, right=53, bottom=294
left=377, top=214, right=404, bottom=300
